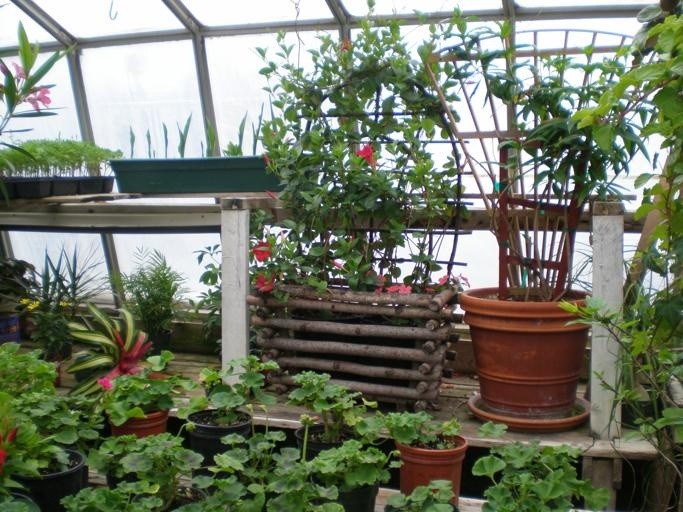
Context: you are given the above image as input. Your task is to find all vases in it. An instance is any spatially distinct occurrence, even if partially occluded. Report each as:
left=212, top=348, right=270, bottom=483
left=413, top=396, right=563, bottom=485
left=281, top=288, right=424, bottom=405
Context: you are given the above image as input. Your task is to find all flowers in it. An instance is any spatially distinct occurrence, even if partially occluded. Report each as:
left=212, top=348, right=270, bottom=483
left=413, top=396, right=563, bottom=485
left=1, top=20, right=80, bottom=133
left=246, top=2, right=491, bottom=298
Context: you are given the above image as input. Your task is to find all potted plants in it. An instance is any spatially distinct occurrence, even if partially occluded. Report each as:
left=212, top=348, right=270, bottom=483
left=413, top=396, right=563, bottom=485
left=2, top=226, right=319, bottom=510
left=288, top=368, right=505, bottom=508
left=1, top=131, right=123, bottom=198
left=108, top=101, right=319, bottom=194
left=429, top=18, right=657, bottom=434
